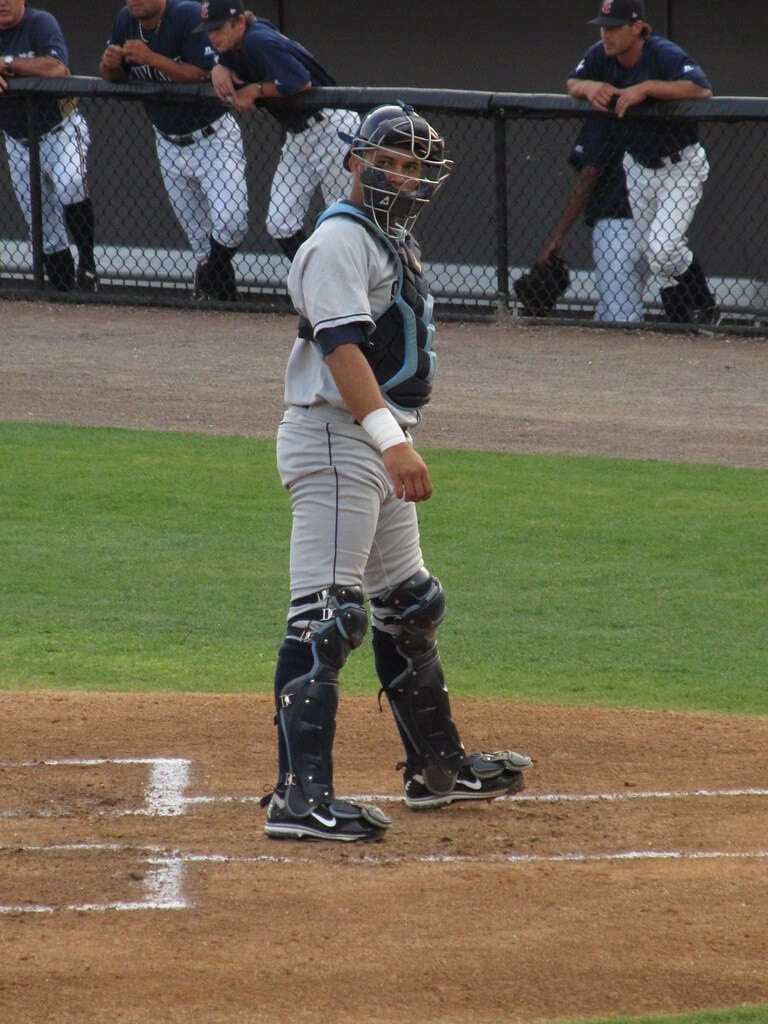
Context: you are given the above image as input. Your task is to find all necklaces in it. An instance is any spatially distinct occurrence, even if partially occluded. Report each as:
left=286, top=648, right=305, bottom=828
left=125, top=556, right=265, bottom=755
left=139, top=18, right=162, bottom=43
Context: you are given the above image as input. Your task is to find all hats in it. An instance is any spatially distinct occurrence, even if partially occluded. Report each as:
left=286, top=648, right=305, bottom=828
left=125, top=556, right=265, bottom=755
left=585, top=0, right=647, bottom=28
left=192, top=0, right=244, bottom=34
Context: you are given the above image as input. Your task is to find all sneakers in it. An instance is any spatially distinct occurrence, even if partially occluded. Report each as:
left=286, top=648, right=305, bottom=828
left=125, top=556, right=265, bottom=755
left=262, top=783, right=391, bottom=844
left=395, top=752, right=527, bottom=808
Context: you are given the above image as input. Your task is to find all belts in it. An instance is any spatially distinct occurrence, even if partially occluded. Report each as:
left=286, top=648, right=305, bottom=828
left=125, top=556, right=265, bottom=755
left=158, top=126, right=215, bottom=148
left=289, top=112, right=325, bottom=134
left=10, top=125, right=62, bottom=147
left=627, top=149, right=679, bottom=169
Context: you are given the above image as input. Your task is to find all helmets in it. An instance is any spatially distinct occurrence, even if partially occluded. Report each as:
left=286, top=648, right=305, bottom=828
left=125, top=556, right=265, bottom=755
left=339, top=100, right=444, bottom=217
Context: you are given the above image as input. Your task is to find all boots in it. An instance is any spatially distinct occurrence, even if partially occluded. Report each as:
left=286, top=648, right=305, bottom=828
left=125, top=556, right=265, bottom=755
left=660, top=254, right=723, bottom=333
left=41, top=198, right=99, bottom=292
left=278, top=230, right=308, bottom=265
left=193, top=231, right=243, bottom=301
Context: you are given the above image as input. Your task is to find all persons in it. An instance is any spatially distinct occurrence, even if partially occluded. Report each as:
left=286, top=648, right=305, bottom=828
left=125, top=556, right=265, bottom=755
left=192, top=0, right=365, bottom=263
left=533, top=119, right=651, bottom=322
left=0, top=0, right=100, bottom=294
left=258, top=103, right=532, bottom=844
left=567, top=0, right=722, bottom=332
left=99, top=0, right=248, bottom=301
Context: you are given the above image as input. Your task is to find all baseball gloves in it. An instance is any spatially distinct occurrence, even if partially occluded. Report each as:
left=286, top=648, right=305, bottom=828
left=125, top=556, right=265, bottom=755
left=515, top=253, right=570, bottom=313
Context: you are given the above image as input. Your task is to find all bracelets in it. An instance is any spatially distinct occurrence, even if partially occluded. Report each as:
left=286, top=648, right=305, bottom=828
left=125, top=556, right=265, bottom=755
left=258, top=82, right=266, bottom=99
left=362, top=408, right=409, bottom=454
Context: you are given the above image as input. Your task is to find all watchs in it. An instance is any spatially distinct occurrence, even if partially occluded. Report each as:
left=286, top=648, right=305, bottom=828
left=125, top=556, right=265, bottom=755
left=4, top=55, right=14, bottom=73
